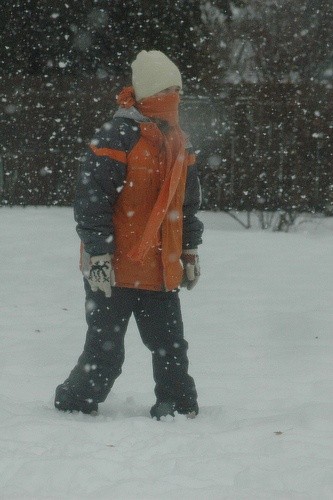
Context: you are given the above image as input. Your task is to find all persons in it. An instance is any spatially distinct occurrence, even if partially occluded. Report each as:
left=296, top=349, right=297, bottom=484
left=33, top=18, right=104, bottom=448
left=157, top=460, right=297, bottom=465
left=54, top=49, right=204, bottom=420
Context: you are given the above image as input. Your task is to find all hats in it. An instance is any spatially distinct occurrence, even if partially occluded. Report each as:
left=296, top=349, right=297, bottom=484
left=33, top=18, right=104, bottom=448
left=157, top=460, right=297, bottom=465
left=130, top=50, right=182, bottom=102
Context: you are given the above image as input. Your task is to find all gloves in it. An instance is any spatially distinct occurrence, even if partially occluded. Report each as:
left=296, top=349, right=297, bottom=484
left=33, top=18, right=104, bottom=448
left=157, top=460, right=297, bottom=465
left=180, top=249, right=201, bottom=290
left=84, top=254, right=116, bottom=298
